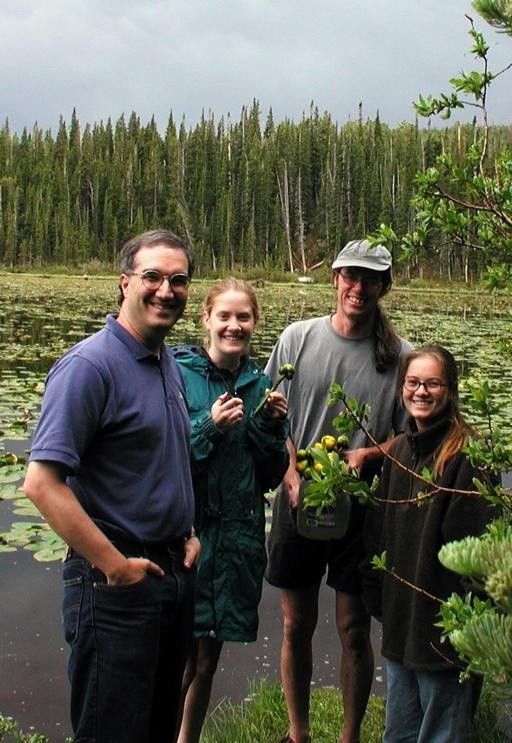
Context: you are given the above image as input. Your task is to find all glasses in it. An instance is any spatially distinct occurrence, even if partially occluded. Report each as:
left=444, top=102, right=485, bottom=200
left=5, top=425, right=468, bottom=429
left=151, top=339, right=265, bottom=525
left=127, top=269, right=191, bottom=294
left=403, top=377, right=448, bottom=393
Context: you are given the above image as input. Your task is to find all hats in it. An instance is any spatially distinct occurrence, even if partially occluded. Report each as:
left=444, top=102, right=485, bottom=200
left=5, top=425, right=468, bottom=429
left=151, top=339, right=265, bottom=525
left=331, top=240, right=393, bottom=272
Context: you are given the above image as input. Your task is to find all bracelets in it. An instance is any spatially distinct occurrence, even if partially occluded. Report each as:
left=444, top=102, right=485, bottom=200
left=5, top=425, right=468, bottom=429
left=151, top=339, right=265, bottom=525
left=179, top=531, right=197, bottom=543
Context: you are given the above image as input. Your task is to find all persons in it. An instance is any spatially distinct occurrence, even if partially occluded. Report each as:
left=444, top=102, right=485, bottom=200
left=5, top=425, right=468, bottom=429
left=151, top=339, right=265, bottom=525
left=168, top=276, right=287, bottom=743
left=377, top=346, right=501, bottom=743
left=24, top=230, right=202, bottom=743
left=262, top=240, right=416, bottom=743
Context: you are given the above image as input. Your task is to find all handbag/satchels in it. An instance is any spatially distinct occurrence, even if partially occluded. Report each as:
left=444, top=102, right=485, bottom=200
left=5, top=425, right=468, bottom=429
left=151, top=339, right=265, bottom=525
left=296, top=479, right=352, bottom=542
